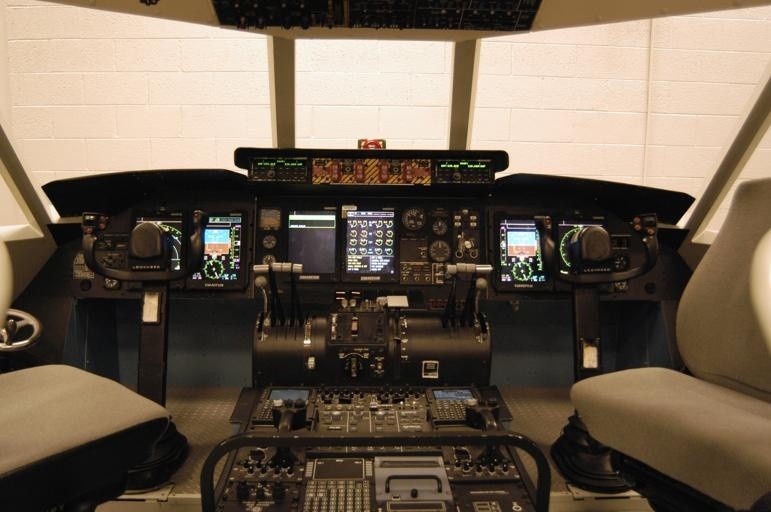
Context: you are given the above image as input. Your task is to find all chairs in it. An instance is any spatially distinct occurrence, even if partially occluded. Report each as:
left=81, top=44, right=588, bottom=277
left=0, top=239, right=172, bottom=512
left=570, top=178, right=771, bottom=512
